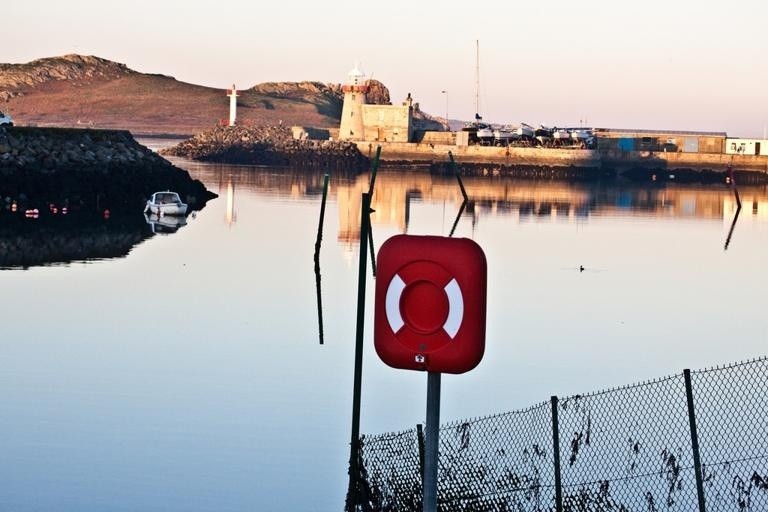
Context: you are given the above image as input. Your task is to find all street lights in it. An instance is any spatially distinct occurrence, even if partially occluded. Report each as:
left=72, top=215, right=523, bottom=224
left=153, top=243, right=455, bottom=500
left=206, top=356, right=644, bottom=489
left=441, top=90, right=449, bottom=132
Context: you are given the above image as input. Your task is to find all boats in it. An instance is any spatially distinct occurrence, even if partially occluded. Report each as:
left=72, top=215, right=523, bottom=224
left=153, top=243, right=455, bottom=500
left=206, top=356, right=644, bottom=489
left=142, top=189, right=189, bottom=218
left=463, top=120, right=593, bottom=147
